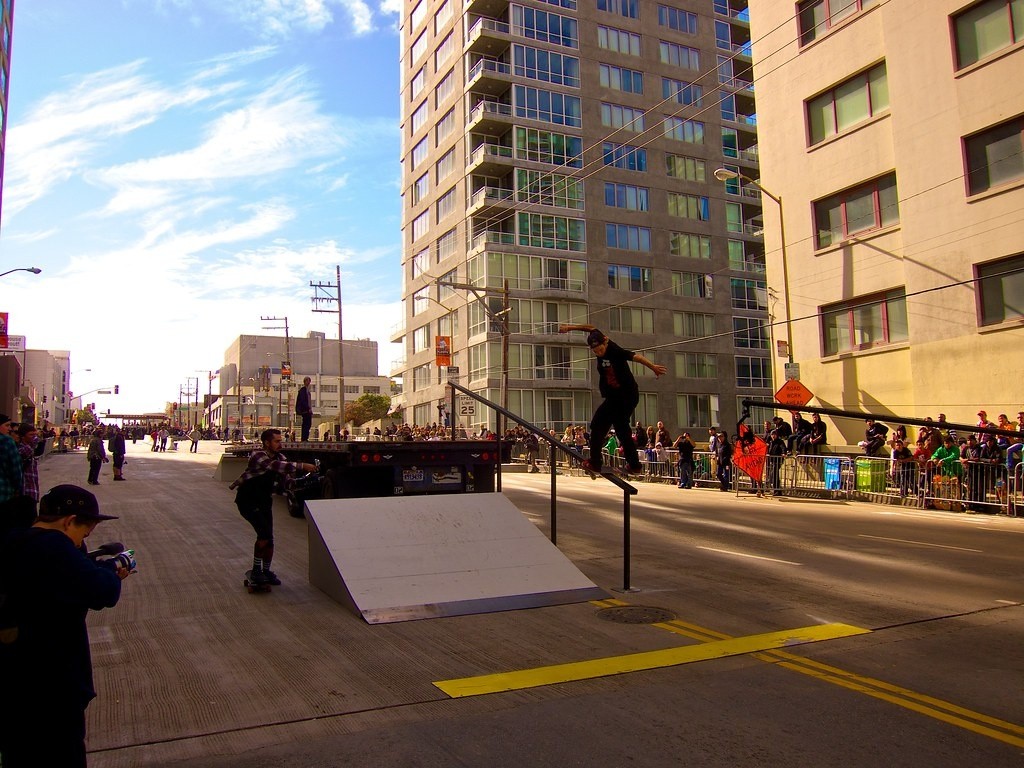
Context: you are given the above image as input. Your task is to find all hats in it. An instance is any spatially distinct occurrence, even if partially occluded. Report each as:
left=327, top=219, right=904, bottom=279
left=717, top=433, right=724, bottom=437
left=0, top=414, right=11, bottom=424
left=587, top=328, right=609, bottom=349
left=38, top=485, right=119, bottom=524
left=770, top=429, right=778, bottom=435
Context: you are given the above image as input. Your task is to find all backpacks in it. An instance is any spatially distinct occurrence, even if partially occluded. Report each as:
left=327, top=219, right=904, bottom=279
left=108, top=435, right=123, bottom=452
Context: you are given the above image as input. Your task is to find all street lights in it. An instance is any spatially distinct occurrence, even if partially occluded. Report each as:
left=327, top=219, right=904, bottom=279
left=414, top=295, right=454, bottom=381
left=712, top=168, right=799, bottom=456
left=266, top=352, right=291, bottom=433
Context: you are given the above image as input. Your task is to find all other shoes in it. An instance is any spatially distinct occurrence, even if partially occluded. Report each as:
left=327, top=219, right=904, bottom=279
left=93, top=480, right=100, bottom=485
left=582, top=458, right=601, bottom=476
left=265, top=571, right=281, bottom=585
left=114, top=476, right=125, bottom=480
left=678, top=486, right=684, bottom=489
left=684, top=486, right=691, bottom=489
left=251, top=572, right=268, bottom=583
left=625, top=463, right=642, bottom=475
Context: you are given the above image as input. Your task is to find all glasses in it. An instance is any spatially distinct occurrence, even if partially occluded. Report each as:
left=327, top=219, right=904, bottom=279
left=11, top=429, right=19, bottom=435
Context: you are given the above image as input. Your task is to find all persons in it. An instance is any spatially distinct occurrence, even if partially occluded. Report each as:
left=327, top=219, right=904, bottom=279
left=292, top=430, right=296, bottom=441
left=112, top=429, right=126, bottom=481
left=295, top=376, right=314, bottom=442
left=864, top=419, right=889, bottom=455
left=384, top=422, right=468, bottom=442
left=0, top=414, right=221, bottom=768
left=472, top=420, right=673, bottom=474
left=254, top=430, right=258, bottom=437
left=747, top=413, right=826, bottom=496
left=189, top=425, right=201, bottom=453
left=709, top=426, right=718, bottom=479
left=228, top=429, right=321, bottom=585
left=223, top=426, right=240, bottom=443
left=158, top=426, right=169, bottom=451
left=716, top=430, right=732, bottom=491
left=365, top=428, right=370, bottom=434
left=344, top=428, right=349, bottom=441
left=892, top=410, right=1024, bottom=502
left=87, top=430, right=106, bottom=484
left=324, top=430, right=330, bottom=441
left=673, top=433, right=695, bottom=489
left=373, top=427, right=381, bottom=435
left=150, top=429, right=158, bottom=450
left=284, top=430, right=289, bottom=441
left=559, top=324, right=668, bottom=475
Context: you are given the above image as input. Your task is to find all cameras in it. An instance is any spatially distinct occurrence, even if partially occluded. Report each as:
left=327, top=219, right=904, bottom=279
left=680, top=435, right=686, bottom=439
left=87, top=542, right=138, bottom=576
left=285, top=469, right=330, bottom=490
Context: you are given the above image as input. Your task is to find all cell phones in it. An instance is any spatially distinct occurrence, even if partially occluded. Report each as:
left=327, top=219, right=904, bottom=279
left=37, top=430, right=43, bottom=442
left=892, top=432, right=895, bottom=441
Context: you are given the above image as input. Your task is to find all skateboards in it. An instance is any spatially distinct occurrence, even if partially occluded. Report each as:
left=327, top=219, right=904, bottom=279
left=580, top=459, right=644, bottom=481
left=243, top=570, right=272, bottom=594
